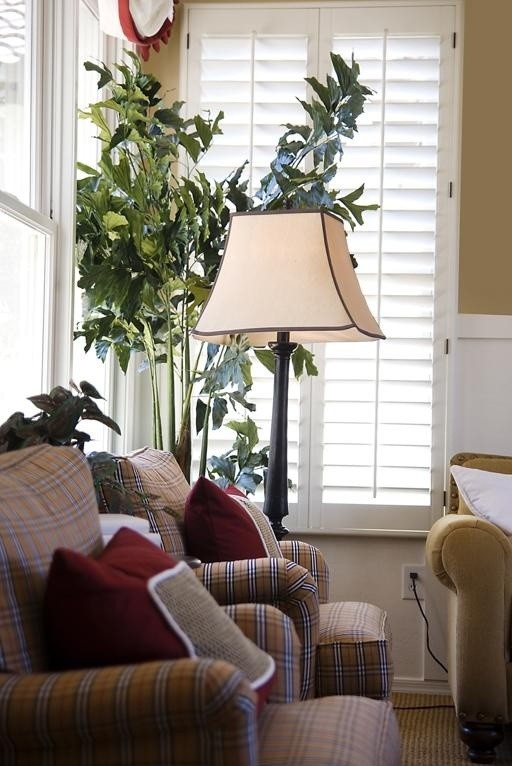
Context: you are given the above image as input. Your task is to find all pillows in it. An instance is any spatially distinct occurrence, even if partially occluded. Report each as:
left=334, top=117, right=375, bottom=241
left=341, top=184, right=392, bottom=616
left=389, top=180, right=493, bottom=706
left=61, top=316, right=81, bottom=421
left=37, top=527, right=275, bottom=715
left=448, top=466, right=512, bottom=536
left=182, top=476, right=284, bottom=562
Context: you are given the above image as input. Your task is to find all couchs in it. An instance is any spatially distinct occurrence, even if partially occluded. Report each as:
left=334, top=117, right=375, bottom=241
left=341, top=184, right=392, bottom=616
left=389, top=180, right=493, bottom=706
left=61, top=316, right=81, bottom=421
left=0, top=443, right=403, bottom=765
left=425, top=452, right=512, bottom=764
left=89, top=444, right=395, bottom=697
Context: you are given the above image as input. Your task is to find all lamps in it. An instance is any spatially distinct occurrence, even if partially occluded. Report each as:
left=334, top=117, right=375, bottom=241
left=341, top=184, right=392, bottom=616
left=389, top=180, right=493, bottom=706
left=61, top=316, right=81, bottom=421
left=191, top=210, right=385, bottom=541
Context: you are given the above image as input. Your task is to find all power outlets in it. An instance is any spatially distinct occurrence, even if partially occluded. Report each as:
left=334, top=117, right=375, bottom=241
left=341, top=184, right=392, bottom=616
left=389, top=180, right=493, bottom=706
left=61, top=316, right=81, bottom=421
left=401, top=563, right=425, bottom=600
